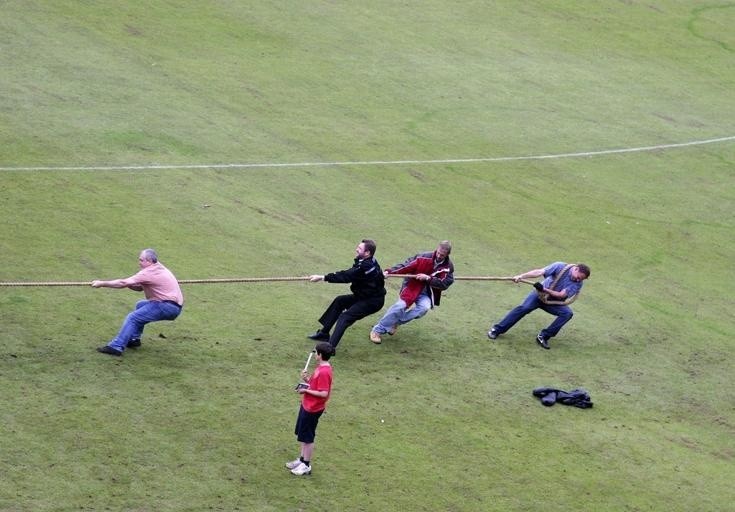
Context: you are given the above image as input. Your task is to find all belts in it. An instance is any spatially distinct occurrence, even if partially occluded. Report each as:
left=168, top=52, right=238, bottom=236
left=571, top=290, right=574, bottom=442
left=164, top=299, right=182, bottom=308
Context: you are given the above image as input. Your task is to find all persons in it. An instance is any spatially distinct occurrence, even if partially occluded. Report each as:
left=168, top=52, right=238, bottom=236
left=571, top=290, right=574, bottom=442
left=90, top=248, right=183, bottom=358
left=368, top=240, right=454, bottom=345
left=284, top=342, right=333, bottom=476
left=487, top=261, right=590, bottom=349
left=307, top=238, right=387, bottom=348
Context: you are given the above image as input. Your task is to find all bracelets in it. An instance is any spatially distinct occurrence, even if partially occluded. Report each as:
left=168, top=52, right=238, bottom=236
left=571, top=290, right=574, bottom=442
left=425, top=275, right=429, bottom=281
left=321, top=275, right=325, bottom=281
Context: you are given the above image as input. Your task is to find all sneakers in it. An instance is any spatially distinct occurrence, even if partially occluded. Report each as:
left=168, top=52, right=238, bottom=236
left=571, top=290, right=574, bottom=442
left=285, top=458, right=302, bottom=469
left=370, top=332, right=381, bottom=344
left=536, top=334, right=550, bottom=349
left=388, top=325, right=397, bottom=335
left=307, top=330, right=330, bottom=341
left=313, top=349, right=336, bottom=356
left=97, top=346, right=121, bottom=356
left=487, top=328, right=497, bottom=339
left=126, top=339, right=141, bottom=348
left=290, top=463, right=311, bottom=476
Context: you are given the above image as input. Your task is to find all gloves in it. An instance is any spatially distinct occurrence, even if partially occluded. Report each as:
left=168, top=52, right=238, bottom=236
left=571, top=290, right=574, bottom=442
left=534, top=282, right=543, bottom=291
